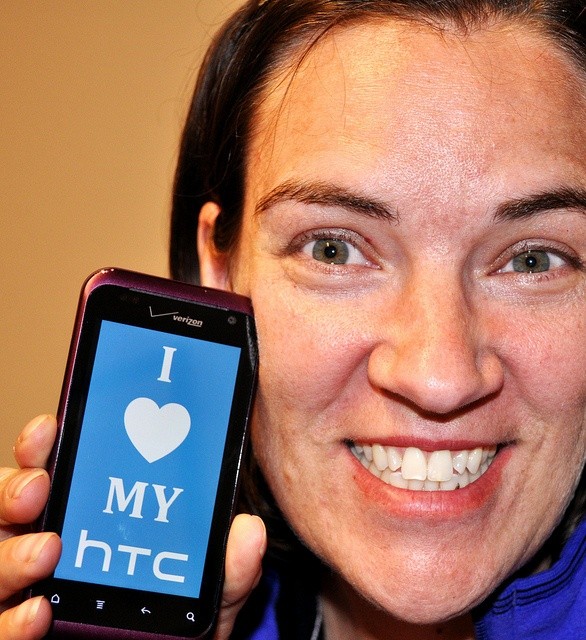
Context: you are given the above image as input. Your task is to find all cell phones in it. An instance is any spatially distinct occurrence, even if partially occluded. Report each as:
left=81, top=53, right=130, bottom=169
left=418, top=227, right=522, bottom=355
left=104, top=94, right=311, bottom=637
left=17, top=267, right=259, bottom=640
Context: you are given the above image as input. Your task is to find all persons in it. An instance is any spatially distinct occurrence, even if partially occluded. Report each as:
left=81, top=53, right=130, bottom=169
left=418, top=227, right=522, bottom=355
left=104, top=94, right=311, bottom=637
left=1, top=0, right=586, bottom=639
